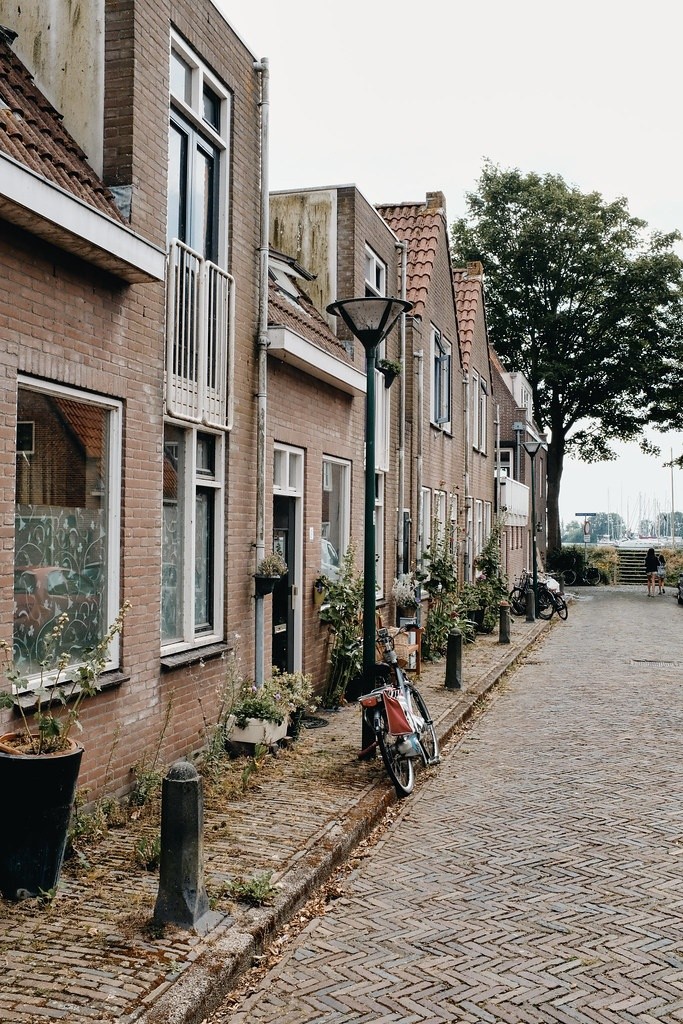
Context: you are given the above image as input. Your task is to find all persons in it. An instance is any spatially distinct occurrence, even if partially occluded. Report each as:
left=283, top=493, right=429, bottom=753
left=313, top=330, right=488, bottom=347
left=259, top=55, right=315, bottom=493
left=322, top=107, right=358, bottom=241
left=645, top=548, right=660, bottom=598
left=657, top=555, right=667, bottom=595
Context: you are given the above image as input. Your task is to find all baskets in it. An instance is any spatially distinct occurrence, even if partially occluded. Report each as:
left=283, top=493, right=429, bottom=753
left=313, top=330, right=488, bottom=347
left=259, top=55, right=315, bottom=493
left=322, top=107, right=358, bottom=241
left=374, top=630, right=410, bottom=671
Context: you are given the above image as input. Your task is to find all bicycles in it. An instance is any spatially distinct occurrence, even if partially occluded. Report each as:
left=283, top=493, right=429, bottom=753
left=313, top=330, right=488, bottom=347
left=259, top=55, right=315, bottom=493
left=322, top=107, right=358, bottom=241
left=559, top=553, right=602, bottom=587
left=344, top=621, right=444, bottom=796
left=509, top=568, right=555, bottom=620
left=536, top=570, right=570, bottom=621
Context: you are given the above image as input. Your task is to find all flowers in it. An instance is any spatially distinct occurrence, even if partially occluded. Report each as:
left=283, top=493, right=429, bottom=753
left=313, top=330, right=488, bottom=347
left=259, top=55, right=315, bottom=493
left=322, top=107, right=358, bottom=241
left=225, top=675, right=295, bottom=729
left=1, top=598, right=135, bottom=754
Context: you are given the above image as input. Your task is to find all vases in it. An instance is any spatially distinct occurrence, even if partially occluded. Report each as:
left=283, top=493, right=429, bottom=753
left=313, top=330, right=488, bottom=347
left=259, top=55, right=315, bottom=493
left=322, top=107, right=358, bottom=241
left=226, top=711, right=288, bottom=746
left=0, top=733, right=84, bottom=905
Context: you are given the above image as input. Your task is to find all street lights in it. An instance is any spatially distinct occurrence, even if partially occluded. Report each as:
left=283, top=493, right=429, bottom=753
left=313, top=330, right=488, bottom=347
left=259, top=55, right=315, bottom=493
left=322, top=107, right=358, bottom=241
left=324, top=292, right=418, bottom=762
left=521, top=439, right=547, bottom=621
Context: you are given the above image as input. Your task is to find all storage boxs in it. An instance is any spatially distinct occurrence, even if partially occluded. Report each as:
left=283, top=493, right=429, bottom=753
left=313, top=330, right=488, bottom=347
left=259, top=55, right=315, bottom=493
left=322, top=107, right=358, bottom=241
left=374, top=625, right=410, bottom=673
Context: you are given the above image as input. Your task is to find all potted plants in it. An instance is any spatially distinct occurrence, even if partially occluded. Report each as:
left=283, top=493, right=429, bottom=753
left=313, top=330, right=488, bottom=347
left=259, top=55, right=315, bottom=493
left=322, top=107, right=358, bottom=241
left=313, top=574, right=333, bottom=610
left=392, top=573, right=424, bottom=619
left=377, top=358, right=402, bottom=389
left=251, top=551, right=288, bottom=598
left=459, top=573, right=491, bottom=635
